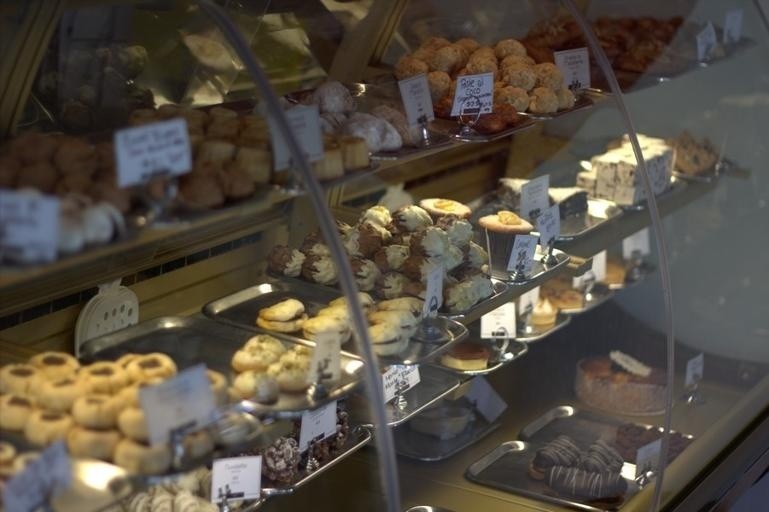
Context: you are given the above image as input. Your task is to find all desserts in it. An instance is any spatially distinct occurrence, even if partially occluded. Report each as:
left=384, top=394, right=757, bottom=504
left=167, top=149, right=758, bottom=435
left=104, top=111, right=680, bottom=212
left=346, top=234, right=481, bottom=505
left=1, top=16, right=727, bottom=512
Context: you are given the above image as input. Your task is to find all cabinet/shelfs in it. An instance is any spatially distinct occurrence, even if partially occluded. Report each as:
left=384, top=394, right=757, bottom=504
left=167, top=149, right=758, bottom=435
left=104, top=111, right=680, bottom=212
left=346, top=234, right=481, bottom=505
left=2, top=1, right=763, bottom=509
